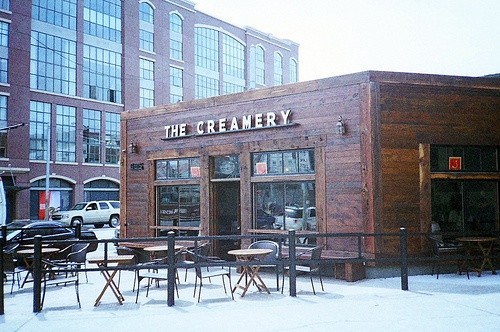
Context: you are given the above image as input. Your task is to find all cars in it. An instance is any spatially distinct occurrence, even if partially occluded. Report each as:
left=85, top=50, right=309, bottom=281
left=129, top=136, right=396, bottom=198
left=257, top=208, right=276, bottom=229
left=0, top=218, right=98, bottom=271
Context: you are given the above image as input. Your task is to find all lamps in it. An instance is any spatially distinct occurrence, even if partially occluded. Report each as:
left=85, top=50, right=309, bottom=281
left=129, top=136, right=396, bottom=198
left=336, top=117, right=347, bottom=136
left=129, top=142, right=137, bottom=154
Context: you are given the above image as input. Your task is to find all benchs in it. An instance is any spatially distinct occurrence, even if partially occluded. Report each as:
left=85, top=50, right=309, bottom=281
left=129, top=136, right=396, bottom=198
left=278, top=246, right=366, bottom=282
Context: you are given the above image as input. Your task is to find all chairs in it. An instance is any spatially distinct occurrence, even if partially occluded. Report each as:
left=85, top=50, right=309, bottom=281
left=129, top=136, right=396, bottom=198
left=184, top=247, right=235, bottom=303
left=2, top=236, right=90, bottom=308
left=281, top=243, right=325, bottom=295
left=245, top=240, right=280, bottom=292
left=425, top=233, right=470, bottom=280
left=115, top=240, right=183, bottom=299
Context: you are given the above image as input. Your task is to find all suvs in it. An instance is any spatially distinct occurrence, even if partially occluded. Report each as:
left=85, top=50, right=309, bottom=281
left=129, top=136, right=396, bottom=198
left=49, top=200, right=120, bottom=230
left=275, top=206, right=316, bottom=231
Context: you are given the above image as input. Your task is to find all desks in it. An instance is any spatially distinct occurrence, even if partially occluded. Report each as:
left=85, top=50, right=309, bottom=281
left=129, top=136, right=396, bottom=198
left=457, top=236, right=498, bottom=277
left=17, top=248, right=59, bottom=288
left=227, top=249, right=274, bottom=297
left=144, top=245, right=185, bottom=287
left=88, top=256, right=134, bottom=307
